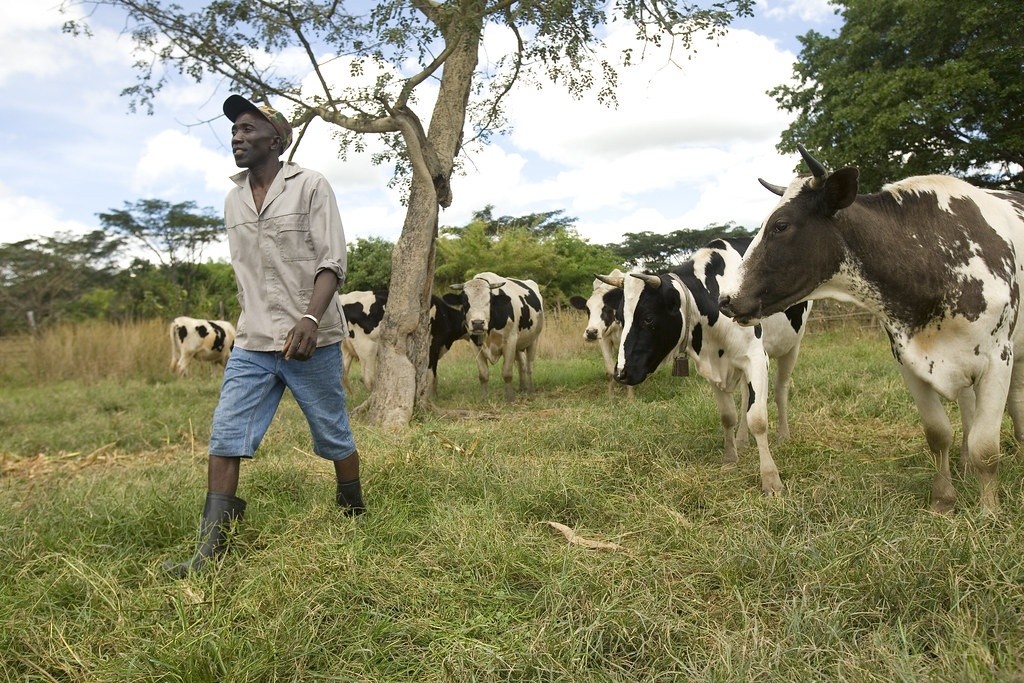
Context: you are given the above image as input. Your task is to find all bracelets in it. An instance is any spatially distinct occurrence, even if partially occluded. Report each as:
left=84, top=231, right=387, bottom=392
left=303, top=314, right=319, bottom=328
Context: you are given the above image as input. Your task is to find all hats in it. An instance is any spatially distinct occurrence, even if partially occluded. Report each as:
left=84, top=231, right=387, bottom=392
left=222, top=94, right=293, bottom=153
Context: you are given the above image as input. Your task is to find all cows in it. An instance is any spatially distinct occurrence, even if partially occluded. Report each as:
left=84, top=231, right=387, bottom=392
left=718, top=143, right=1023, bottom=523
left=170, top=316, right=236, bottom=377
left=338, top=236, right=636, bottom=404
left=593, top=235, right=814, bottom=500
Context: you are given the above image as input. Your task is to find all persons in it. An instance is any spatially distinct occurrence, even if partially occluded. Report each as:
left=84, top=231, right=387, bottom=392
left=158, top=94, right=368, bottom=575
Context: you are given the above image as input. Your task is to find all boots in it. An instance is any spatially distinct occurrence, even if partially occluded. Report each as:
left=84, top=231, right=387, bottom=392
left=162, top=492, right=247, bottom=580
left=336, top=480, right=372, bottom=519
